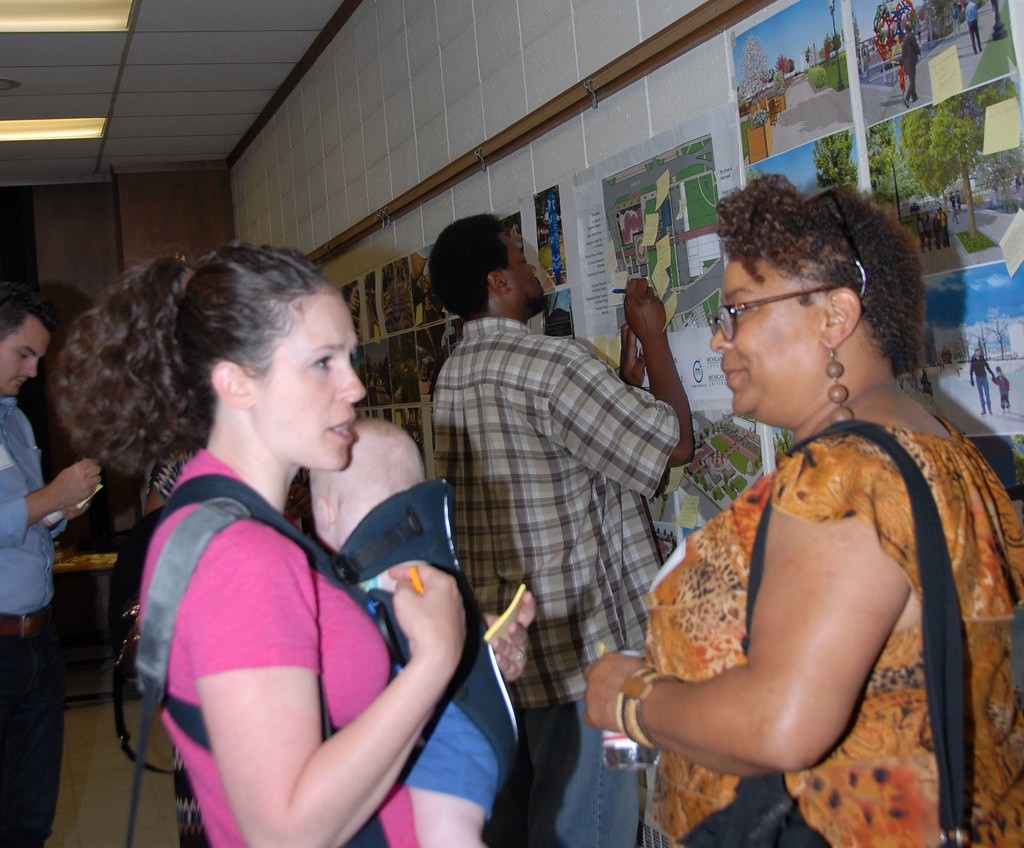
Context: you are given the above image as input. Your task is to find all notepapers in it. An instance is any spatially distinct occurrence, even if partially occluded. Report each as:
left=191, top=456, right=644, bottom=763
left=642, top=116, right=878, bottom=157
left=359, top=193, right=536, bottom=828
left=482, top=583, right=526, bottom=644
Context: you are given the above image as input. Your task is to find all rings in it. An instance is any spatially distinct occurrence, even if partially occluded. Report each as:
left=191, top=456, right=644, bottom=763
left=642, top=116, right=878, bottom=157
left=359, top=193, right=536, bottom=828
left=515, top=649, right=525, bottom=662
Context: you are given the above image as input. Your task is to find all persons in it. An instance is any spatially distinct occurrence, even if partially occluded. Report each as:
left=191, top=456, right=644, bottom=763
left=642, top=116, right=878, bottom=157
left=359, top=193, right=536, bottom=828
left=913, top=189, right=961, bottom=253
left=970, top=347, right=1011, bottom=416
left=903, top=22, right=921, bottom=108
left=992, top=174, right=1021, bottom=194
left=583, top=174, right=1024, bottom=848
left=0, top=242, right=535, bottom=848
left=966, top=0, right=983, bottom=55
left=428, top=213, right=695, bottom=848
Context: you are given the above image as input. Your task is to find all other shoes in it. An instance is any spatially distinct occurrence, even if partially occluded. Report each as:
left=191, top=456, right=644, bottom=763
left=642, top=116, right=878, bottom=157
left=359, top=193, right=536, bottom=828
left=904, top=98, right=910, bottom=108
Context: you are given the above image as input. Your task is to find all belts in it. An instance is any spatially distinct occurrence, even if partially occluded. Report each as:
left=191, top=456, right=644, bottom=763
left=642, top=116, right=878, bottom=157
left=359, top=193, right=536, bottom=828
left=0, top=603, right=55, bottom=639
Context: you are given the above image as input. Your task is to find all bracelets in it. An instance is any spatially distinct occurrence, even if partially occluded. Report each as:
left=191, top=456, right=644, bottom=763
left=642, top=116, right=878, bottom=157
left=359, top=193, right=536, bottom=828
left=617, top=667, right=683, bottom=753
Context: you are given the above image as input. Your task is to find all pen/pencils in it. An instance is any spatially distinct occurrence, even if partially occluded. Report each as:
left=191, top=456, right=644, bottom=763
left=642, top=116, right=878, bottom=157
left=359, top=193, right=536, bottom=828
left=612, top=289, right=626, bottom=294
left=407, top=565, right=425, bottom=592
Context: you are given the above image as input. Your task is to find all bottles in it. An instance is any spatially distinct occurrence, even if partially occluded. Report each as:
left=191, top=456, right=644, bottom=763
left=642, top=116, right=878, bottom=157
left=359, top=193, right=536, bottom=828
left=28, top=509, right=66, bottom=531
left=602, top=650, right=660, bottom=769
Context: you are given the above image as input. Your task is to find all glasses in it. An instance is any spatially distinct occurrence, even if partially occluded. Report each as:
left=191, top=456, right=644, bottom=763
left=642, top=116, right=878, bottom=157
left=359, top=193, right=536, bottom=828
left=710, top=282, right=867, bottom=341
left=800, top=186, right=865, bottom=275
